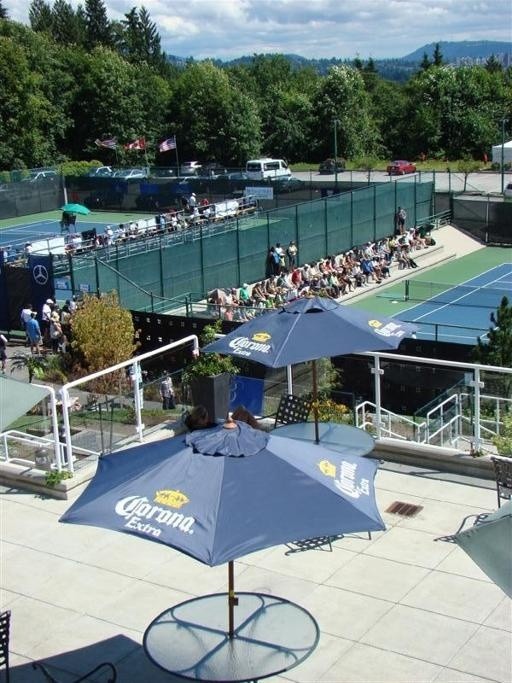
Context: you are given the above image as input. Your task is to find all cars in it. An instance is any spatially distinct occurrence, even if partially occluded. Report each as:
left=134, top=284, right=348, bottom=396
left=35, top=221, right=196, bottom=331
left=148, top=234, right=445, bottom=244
left=87, top=165, right=150, bottom=180
left=181, top=160, right=229, bottom=176
left=388, top=160, right=416, bottom=175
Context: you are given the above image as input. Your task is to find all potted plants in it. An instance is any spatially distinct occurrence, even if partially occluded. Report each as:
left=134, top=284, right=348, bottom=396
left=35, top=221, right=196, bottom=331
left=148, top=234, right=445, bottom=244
left=186, top=318, right=240, bottom=423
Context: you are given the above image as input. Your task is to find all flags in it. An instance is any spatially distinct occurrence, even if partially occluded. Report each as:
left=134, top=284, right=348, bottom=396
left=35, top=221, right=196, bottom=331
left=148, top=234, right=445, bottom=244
left=94, top=135, right=118, bottom=151
left=158, top=134, right=177, bottom=153
left=122, top=135, right=146, bottom=151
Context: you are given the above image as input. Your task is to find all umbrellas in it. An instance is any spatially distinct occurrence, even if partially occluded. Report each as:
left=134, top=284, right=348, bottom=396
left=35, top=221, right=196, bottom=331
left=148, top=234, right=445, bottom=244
left=197, top=287, right=419, bottom=442
left=55, top=409, right=387, bottom=634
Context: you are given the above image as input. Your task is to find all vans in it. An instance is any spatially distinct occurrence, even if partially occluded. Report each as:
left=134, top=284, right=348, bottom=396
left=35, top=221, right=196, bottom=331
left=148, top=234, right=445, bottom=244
left=246, top=157, right=293, bottom=181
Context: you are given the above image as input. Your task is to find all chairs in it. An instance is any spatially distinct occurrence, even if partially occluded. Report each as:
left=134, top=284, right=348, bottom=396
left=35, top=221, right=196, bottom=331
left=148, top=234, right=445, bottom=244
left=274, top=393, right=310, bottom=429
left=492, top=457, right=512, bottom=509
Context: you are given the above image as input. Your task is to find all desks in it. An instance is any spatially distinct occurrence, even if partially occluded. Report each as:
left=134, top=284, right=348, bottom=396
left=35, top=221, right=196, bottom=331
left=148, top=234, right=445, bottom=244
left=139, top=590, right=321, bottom=680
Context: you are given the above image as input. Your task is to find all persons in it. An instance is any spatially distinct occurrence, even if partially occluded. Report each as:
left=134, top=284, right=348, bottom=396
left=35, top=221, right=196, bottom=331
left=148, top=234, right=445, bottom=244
left=159, top=369, right=176, bottom=411
left=133, top=356, right=148, bottom=404
left=58, top=190, right=257, bottom=254
left=1, top=247, right=8, bottom=262
left=23, top=241, right=32, bottom=259
left=21, top=293, right=83, bottom=371
left=0, top=334, right=9, bottom=375
left=6, top=245, right=17, bottom=262
left=205, top=207, right=437, bottom=324
left=13, top=245, right=20, bottom=261
